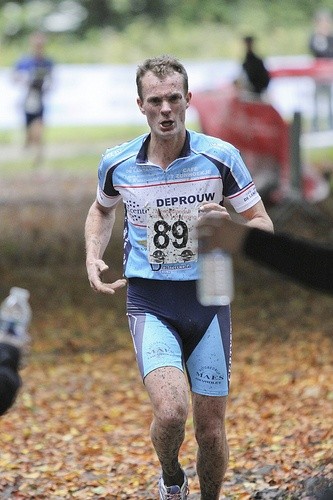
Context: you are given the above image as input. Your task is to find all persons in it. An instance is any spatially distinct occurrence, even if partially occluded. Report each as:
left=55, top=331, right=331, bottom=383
left=0, top=330, right=34, bottom=417
left=233, top=36, right=272, bottom=98
left=84, top=56, right=276, bottom=500
left=193, top=200, right=333, bottom=298
left=11, top=33, right=61, bottom=160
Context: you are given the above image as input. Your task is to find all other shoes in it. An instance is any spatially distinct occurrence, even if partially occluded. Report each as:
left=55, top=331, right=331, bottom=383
left=159, top=472, right=190, bottom=500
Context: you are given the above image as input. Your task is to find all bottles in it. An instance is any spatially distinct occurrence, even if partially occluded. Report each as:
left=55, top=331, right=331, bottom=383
left=0, top=287, right=32, bottom=348
left=197, top=248, right=234, bottom=306
